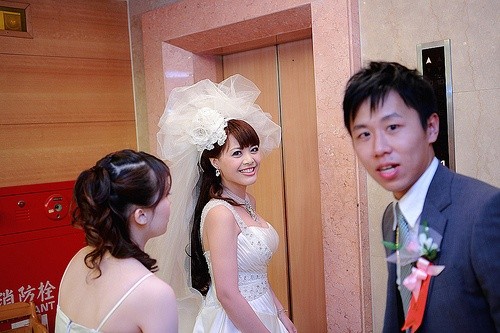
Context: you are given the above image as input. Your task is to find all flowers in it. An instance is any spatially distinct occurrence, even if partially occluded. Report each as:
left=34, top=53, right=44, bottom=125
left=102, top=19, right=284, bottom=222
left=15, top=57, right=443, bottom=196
left=186, top=108, right=229, bottom=151
left=380, top=217, right=445, bottom=301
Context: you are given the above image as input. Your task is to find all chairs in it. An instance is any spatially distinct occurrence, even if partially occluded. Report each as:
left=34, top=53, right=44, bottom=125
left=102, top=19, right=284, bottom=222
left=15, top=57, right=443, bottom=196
left=0, top=301, right=48, bottom=333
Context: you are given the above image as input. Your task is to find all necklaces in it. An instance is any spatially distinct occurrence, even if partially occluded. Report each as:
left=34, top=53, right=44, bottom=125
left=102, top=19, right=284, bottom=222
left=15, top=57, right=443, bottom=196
left=219, top=184, right=258, bottom=221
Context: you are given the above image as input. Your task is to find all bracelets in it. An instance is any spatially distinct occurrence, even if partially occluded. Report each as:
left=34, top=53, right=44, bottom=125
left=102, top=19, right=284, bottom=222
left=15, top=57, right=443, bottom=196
left=277, top=308, right=288, bottom=318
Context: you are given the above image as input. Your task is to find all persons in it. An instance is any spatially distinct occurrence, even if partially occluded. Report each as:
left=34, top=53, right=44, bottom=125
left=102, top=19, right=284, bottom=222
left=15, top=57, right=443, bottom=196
left=154, top=73, right=297, bottom=332
left=55, top=149, right=178, bottom=333
left=343, top=60, right=500, bottom=333
